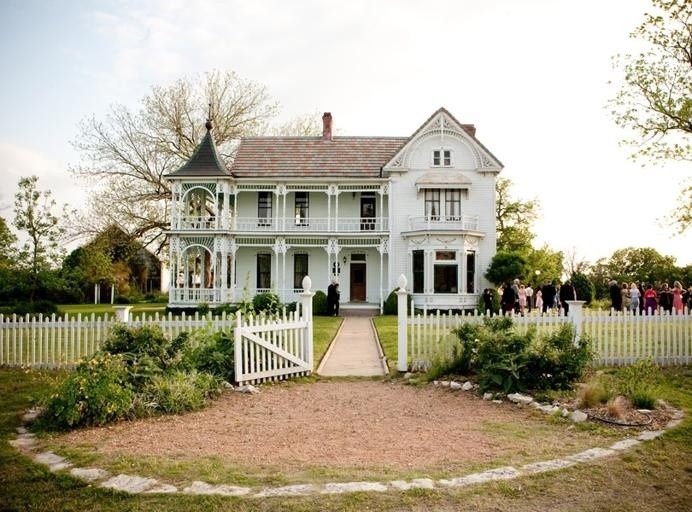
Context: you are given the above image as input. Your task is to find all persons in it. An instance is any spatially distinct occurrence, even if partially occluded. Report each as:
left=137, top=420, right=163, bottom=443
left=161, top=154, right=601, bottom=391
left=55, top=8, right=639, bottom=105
left=332, top=283, right=341, bottom=316
left=482, top=278, right=692, bottom=318
left=325, top=279, right=336, bottom=315
